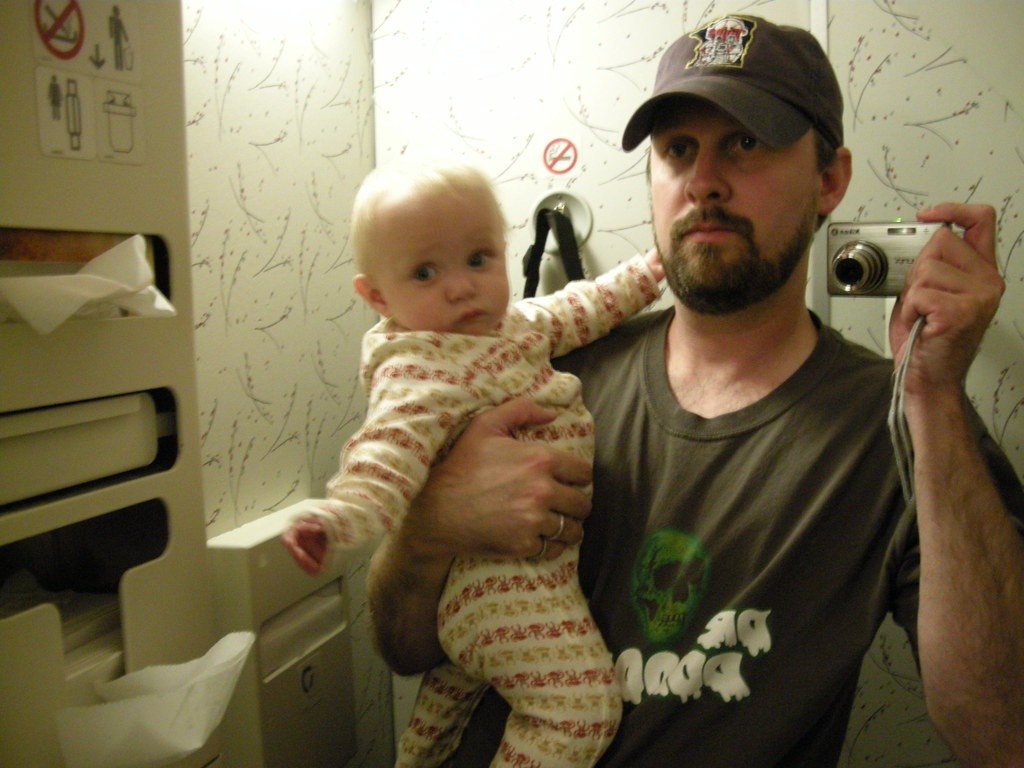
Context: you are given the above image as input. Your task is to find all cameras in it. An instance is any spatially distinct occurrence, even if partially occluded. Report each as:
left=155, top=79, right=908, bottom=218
left=827, top=221, right=950, bottom=298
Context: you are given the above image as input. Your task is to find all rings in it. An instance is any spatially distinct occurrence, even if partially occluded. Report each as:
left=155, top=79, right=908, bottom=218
left=529, top=539, right=548, bottom=560
left=548, top=515, right=566, bottom=540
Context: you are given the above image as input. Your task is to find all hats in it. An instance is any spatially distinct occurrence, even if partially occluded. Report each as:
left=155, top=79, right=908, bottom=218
left=622, top=14, right=844, bottom=152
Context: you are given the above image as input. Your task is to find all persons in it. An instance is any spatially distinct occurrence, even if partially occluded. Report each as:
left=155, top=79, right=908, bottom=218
left=286, top=161, right=666, bottom=768
left=370, top=15, right=1024, bottom=768
